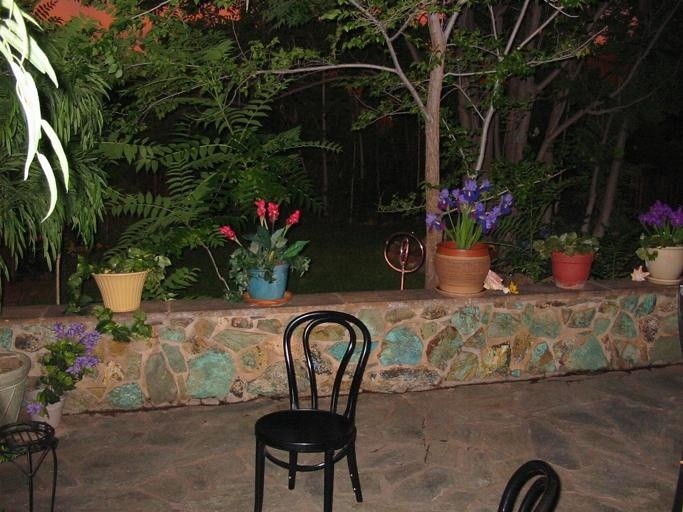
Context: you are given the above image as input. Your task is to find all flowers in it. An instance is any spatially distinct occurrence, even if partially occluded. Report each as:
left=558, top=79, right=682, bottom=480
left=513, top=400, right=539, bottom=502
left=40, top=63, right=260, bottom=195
left=220, top=198, right=311, bottom=295
left=26, top=322, right=104, bottom=419
left=425, top=179, right=513, bottom=249
left=635, top=200, right=683, bottom=261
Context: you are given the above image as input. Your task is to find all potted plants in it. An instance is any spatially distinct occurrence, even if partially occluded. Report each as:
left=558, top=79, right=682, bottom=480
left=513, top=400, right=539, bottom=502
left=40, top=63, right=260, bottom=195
left=532, top=232, right=600, bottom=289
left=63, top=248, right=172, bottom=343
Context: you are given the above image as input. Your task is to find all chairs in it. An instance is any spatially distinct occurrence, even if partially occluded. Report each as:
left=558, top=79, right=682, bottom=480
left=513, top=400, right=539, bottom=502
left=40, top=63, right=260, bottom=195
left=497, top=460, right=561, bottom=512
left=254, top=310, right=371, bottom=512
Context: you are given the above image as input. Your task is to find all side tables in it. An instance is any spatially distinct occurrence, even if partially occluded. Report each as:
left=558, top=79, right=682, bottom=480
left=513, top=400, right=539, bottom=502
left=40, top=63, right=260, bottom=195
left=1, top=422, right=60, bottom=512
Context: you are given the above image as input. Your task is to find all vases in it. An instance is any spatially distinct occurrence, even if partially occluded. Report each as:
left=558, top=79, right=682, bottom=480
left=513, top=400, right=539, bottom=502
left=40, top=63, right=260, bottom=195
left=645, top=247, right=683, bottom=285
left=26, top=389, right=66, bottom=429
left=434, top=241, right=491, bottom=293
left=0, top=351, right=33, bottom=427
left=246, top=263, right=289, bottom=300
